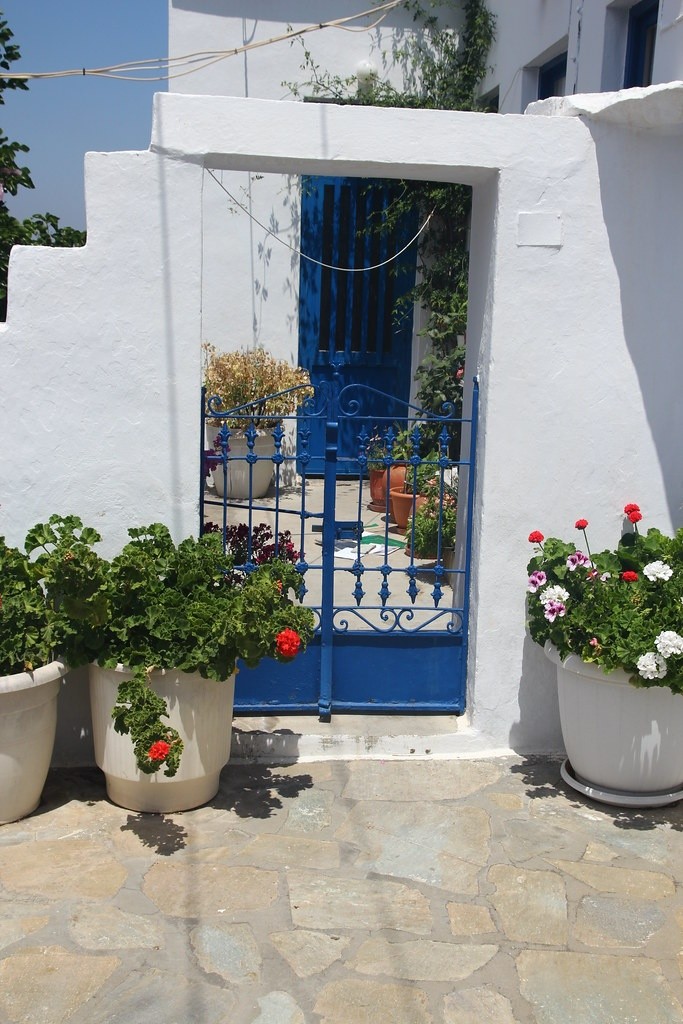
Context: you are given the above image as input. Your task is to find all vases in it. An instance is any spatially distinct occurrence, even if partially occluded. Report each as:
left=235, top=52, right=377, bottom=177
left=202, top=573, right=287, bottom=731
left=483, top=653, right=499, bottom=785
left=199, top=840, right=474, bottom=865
left=442, top=546, right=455, bottom=590
left=89, top=656, right=237, bottom=814
left=382, top=463, right=407, bottom=524
left=544, top=638, right=683, bottom=809
left=367, top=468, right=387, bottom=513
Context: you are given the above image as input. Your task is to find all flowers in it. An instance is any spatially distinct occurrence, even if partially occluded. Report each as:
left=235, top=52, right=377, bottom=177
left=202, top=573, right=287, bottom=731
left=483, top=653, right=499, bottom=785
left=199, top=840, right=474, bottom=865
left=366, top=421, right=405, bottom=470
left=404, top=478, right=458, bottom=559
left=83, top=523, right=315, bottom=778
left=527, top=505, right=683, bottom=696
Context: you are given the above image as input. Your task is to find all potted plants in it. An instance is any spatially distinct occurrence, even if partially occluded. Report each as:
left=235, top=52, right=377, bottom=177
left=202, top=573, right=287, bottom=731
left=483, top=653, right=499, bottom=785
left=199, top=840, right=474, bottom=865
left=390, top=428, right=441, bottom=534
left=0, top=514, right=111, bottom=825
left=202, top=343, right=314, bottom=499
